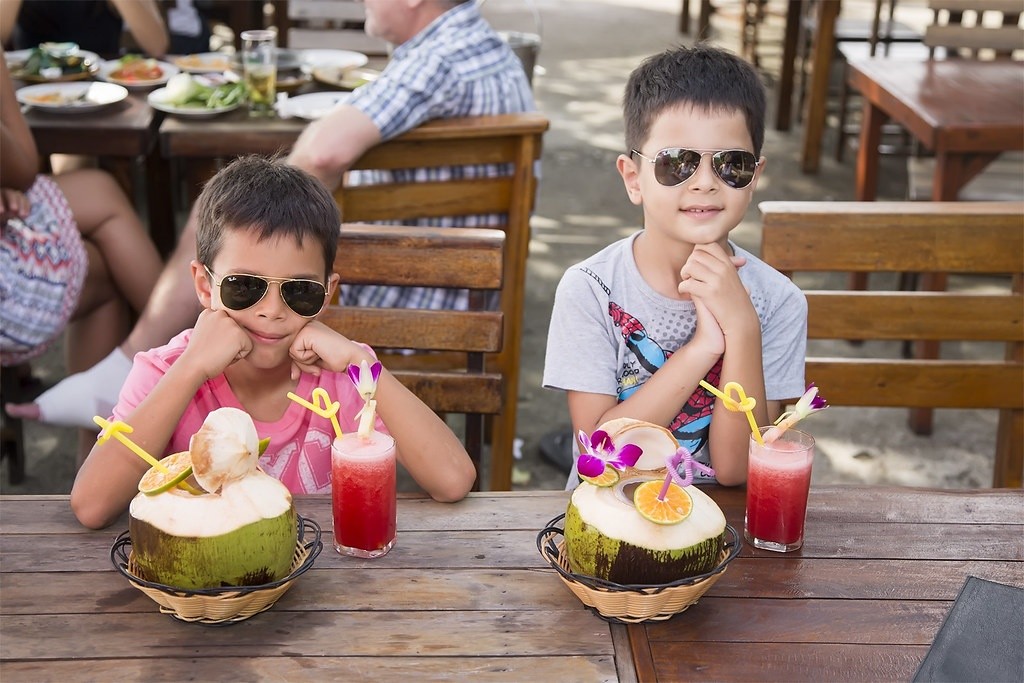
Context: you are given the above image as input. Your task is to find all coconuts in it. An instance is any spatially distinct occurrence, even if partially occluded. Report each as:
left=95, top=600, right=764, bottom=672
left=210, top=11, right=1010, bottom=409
left=562, top=416, right=727, bottom=585
left=128, top=407, right=297, bottom=590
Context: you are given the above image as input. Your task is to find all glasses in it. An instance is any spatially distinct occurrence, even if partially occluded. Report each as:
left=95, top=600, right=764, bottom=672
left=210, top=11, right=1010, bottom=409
left=202, top=263, right=332, bottom=319
left=629, top=147, right=759, bottom=190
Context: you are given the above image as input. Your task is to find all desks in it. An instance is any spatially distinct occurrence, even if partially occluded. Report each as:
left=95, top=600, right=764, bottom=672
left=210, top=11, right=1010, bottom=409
left=0, top=56, right=1024, bottom=683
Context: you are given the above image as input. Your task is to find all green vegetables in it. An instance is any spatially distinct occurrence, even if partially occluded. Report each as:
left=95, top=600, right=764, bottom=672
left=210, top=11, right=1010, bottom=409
left=173, top=80, right=245, bottom=108
left=15, top=49, right=89, bottom=76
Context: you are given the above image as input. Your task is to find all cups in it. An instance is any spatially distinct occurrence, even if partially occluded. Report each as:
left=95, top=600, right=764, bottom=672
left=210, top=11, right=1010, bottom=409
left=241, top=30, right=277, bottom=119
left=743, top=425, right=815, bottom=553
left=330, top=430, right=398, bottom=559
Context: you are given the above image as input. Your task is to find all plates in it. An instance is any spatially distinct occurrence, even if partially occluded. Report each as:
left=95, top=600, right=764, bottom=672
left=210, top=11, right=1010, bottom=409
left=15, top=83, right=128, bottom=112
left=300, top=49, right=369, bottom=68
left=7, top=64, right=100, bottom=84
left=147, top=87, right=241, bottom=118
left=312, top=66, right=382, bottom=89
left=224, top=70, right=311, bottom=90
left=177, top=53, right=232, bottom=72
left=286, top=91, right=354, bottom=121
left=94, top=59, right=177, bottom=89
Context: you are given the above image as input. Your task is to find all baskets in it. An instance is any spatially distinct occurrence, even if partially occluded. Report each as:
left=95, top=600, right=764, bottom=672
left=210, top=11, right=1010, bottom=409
left=536, top=513, right=740, bottom=625
left=110, top=513, right=324, bottom=628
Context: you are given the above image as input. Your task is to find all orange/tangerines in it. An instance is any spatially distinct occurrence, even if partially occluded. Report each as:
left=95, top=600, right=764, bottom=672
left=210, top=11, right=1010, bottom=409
left=137, top=451, right=194, bottom=496
left=579, top=462, right=620, bottom=488
left=258, top=436, right=271, bottom=458
left=633, top=479, right=693, bottom=524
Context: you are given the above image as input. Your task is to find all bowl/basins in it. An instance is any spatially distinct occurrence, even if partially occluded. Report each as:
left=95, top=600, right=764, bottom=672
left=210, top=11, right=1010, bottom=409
left=229, top=50, right=300, bottom=80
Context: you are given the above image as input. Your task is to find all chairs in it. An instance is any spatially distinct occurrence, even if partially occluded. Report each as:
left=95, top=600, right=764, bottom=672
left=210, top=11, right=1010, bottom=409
left=164, top=0, right=1024, bottom=491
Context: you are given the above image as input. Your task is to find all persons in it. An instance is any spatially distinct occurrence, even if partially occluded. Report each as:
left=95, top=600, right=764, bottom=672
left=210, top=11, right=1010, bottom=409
left=673, top=152, right=697, bottom=181
left=70, top=154, right=476, bottom=530
left=5, top=0, right=540, bottom=432
left=718, top=153, right=740, bottom=182
left=0, top=0, right=170, bottom=176
left=0, top=42, right=163, bottom=473
left=541, top=39, right=808, bottom=487
